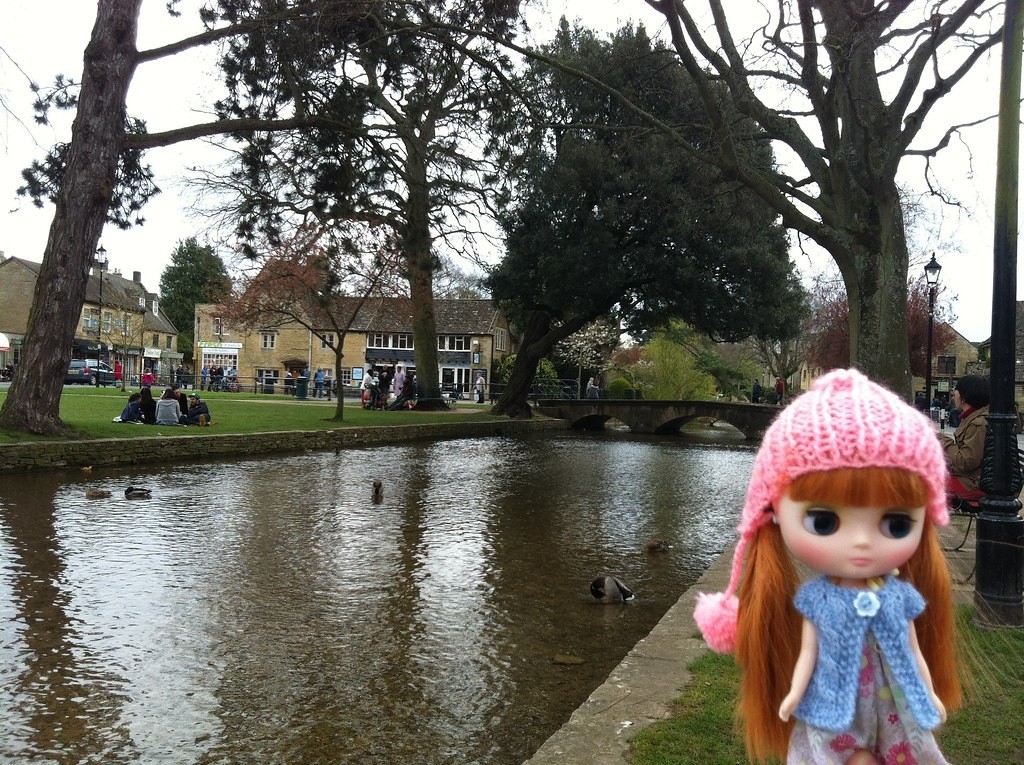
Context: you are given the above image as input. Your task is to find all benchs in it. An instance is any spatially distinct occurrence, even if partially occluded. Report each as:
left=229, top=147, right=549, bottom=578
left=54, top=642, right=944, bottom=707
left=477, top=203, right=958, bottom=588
left=944, top=447, right=1024, bottom=585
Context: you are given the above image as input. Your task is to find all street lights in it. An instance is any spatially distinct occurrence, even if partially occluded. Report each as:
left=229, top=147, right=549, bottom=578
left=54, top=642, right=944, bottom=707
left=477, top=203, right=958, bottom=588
left=97, top=244, right=107, bottom=387
left=924, top=252, right=942, bottom=412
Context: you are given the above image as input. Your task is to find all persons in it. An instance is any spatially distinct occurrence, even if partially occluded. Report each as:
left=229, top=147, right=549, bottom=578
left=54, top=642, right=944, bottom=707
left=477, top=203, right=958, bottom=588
left=695, top=368, right=963, bottom=765
left=752, top=379, right=762, bottom=403
left=283, top=365, right=324, bottom=399
left=473, top=372, right=485, bottom=403
left=112, top=359, right=238, bottom=427
left=361, top=365, right=418, bottom=411
left=915, top=392, right=956, bottom=410
left=587, top=382, right=599, bottom=399
left=5, top=361, right=15, bottom=381
left=775, top=377, right=784, bottom=405
left=586, top=377, right=594, bottom=394
left=935, top=375, right=992, bottom=502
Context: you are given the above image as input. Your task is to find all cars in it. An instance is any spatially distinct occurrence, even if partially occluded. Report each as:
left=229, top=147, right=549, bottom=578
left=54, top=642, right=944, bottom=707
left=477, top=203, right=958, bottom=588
left=65, top=358, right=115, bottom=386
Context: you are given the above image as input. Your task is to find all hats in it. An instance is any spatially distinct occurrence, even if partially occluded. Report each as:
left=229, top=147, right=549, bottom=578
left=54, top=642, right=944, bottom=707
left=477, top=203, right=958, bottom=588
left=188, top=391, right=200, bottom=399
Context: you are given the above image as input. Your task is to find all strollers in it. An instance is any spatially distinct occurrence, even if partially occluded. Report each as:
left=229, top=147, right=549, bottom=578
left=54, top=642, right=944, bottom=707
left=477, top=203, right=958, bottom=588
left=364, top=383, right=389, bottom=411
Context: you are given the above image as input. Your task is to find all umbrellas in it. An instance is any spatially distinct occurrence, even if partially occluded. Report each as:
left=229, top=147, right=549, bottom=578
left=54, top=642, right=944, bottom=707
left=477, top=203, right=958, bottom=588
left=0, top=333, right=9, bottom=351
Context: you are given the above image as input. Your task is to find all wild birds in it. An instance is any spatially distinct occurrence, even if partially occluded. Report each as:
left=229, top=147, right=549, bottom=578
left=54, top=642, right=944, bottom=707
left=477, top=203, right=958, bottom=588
left=372, top=480, right=384, bottom=494
left=82, top=466, right=92, bottom=476
left=125, top=486, right=152, bottom=496
left=591, top=575, right=635, bottom=603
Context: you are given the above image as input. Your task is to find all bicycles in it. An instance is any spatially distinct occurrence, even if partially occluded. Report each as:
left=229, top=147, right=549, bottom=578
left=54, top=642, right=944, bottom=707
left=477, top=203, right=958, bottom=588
left=449, top=389, right=464, bottom=400
left=213, top=379, right=239, bottom=392
